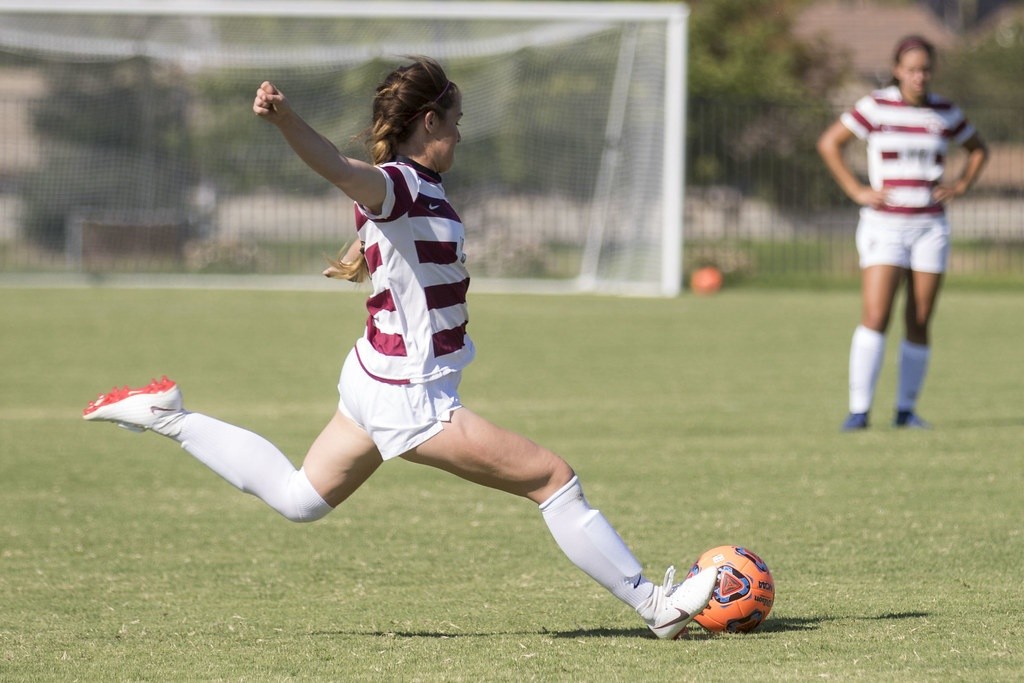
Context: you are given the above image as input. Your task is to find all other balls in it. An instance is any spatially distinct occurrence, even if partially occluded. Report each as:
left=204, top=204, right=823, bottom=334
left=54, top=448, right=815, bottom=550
left=689, top=267, right=724, bottom=294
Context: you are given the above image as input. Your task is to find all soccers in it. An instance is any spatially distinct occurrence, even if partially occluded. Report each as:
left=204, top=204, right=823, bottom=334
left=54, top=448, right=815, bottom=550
left=685, top=543, right=775, bottom=636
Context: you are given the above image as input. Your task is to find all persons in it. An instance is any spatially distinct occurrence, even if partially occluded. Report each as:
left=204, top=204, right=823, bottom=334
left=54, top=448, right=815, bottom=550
left=81, top=57, right=718, bottom=640
left=816, top=35, right=990, bottom=430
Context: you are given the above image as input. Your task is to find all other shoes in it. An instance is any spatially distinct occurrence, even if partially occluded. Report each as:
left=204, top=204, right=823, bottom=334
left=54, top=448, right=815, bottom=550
left=894, top=411, right=935, bottom=430
left=841, top=413, right=867, bottom=432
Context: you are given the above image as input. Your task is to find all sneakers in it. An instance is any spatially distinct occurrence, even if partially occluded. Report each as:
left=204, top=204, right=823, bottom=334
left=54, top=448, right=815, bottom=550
left=648, top=565, right=718, bottom=639
left=83, top=375, right=183, bottom=431
